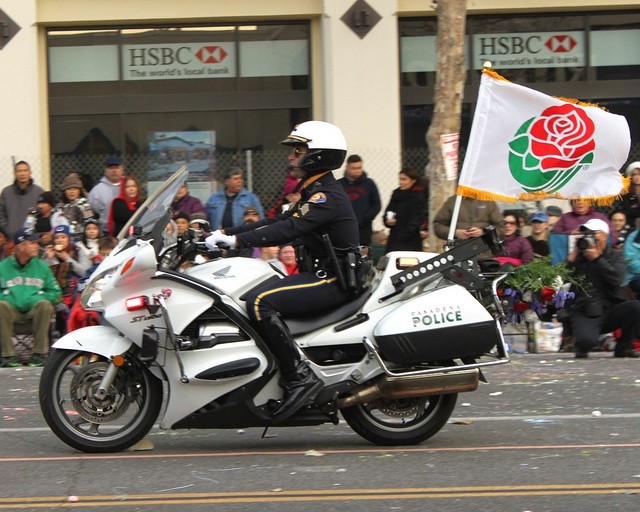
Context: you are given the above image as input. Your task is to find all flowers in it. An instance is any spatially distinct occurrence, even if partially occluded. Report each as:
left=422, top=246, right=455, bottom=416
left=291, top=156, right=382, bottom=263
left=496, top=254, right=596, bottom=321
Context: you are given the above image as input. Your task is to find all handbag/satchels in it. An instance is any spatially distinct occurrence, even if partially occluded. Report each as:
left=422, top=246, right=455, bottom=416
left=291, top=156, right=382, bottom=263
left=49, top=262, right=72, bottom=288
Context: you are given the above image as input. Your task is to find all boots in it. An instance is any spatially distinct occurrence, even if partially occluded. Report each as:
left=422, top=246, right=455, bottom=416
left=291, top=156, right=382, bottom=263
left=253, top=313, right=325, bottom=425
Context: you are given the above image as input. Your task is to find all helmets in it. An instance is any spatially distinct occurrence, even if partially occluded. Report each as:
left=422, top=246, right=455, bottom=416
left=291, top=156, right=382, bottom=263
left=280, top=120, right=348, bottom=173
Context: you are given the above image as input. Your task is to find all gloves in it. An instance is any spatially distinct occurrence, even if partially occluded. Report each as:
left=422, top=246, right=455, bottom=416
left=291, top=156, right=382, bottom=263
left=205, top=234, right=237, bottom=252
left=210, top=229, right=222, bottom=235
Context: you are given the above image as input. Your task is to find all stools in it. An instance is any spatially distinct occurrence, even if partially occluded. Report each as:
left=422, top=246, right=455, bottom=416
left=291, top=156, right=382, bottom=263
left=11, top=317, right=59, bottom=366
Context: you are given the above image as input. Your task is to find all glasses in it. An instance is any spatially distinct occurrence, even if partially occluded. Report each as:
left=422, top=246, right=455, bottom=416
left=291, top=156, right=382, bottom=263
left=505, top=221, right=516, bottom=225
left=292, top=147, right=307, bottom=157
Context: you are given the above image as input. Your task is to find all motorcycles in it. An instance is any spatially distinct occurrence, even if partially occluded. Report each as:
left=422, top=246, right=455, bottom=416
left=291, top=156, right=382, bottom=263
left=38, top=165, right=512, bottom=452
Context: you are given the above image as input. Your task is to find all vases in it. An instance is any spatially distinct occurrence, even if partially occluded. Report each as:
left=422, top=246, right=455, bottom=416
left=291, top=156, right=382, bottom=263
left=529, top=320, right=563, bottom=352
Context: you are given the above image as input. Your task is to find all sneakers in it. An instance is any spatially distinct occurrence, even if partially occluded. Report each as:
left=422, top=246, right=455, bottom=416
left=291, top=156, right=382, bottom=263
left=576, top=347, right=587, bottom=358
left=27, top=353, right=48, bottom=367
left=0, top=356, right=23, bottom=367
left=615, top=345, right=640, bottom=357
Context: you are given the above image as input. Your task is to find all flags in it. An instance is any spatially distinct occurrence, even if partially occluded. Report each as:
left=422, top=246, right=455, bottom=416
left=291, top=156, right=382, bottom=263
left=455, top=69, right=632, bottom=204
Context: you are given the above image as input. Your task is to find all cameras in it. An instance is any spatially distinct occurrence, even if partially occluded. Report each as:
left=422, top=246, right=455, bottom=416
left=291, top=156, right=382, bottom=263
left=577, top=235, right=599, bottom=250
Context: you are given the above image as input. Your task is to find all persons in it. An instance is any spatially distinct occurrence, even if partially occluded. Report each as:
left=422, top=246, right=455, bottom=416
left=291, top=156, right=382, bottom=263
left=335, top=155, right=381, bottom=255
left=170, top=181, right=208, bottom=219
left=281, top=245, right=300, bottom=275
left=244, top=206, right=260, bottom=258
left=0, top=230, right=14, bottom=260
left=0, top=160, right=46, bottom=238
left=499, top=209, right=534, bottom=265
left=75, top=235, right=115, bottom=294
left=12, top=191, right=56, bottom=245
left=625, top=162, right=640, bottom=197
left=41, top=225, right=91, bottom=336
left=568, top=219, right=639, bottom=358
left=86, top=156, right=125, bottom=238
left=189, top=211, right=210, bottom=229
left=553, top=200, right=618, bottom=248
left=52, top=172, right=97, bottom=234
left=545, top=205, right=561, bottom=235
left=74, top=219, right=101, bottom=270
left=173, top=211, right=189, bottom=236
left=204, top=121, right=361, bottom=420
left=383, top=167, right=429, bottom=255
left=609, top=210, right=635, bottom=248
left=256, top=245, right=288, bottom=278
left=109, top=172, right=144, bottom=247
left=1, top=228, right=63, bottom=367
left=204, top=166, right=268, bottom=257
left=525, top=212, right=551, bottom=256
left=433, top=194, right=505, bottom=256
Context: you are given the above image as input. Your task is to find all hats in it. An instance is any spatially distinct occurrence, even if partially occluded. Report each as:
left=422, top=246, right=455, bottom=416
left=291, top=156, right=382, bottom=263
left=174, top=212, right=189, bottom=220
left=60, top=173, right=83, bottom=191
left=624, top=161, right=640, bottom=176
left=14, top=228, right=42, bottom=244
left=580, top=218, right=610, bottom=234
left=547, top=206, right=561, bottom=216
left=103, top=157, right=122, bottom=166
left=37, top=192, right=58, bottom=206
left=189, top=214, right=209, bottom=225
left=55, top=225, right=68, bottom=233
left=530, top=211, right=547, bottom=222
